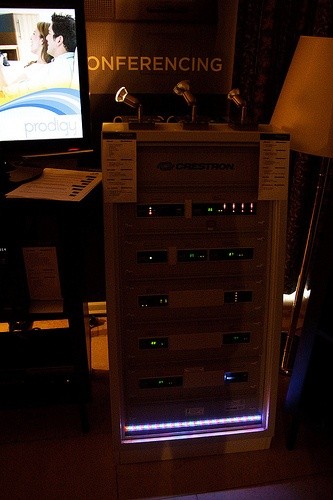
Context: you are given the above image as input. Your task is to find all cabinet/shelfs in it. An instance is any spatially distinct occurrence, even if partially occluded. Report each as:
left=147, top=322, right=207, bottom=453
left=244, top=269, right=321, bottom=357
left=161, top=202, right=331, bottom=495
left=100, top=122, right=292, bottom=465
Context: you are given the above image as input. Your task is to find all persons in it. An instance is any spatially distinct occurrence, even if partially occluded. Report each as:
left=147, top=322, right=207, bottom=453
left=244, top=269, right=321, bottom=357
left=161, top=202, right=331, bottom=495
left=0, top=12, right=77, bottom=96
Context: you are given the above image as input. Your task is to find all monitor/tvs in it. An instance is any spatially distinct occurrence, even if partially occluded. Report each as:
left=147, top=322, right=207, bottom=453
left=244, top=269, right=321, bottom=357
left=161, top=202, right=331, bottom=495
left=1, top=0, right=92, bottom=156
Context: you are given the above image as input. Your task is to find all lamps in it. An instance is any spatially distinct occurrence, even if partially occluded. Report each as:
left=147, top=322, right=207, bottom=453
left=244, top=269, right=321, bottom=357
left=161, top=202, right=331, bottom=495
left=267, top=33, right=332, bottom=378
left=227, top=88, right=259, bottom=132
left=172, top=79, right=208, bottom=130
left=116, top=85, right=157, bottom=129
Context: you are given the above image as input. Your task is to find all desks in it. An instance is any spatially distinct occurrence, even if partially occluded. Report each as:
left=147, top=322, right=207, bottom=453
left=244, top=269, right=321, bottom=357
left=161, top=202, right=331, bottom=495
left=1, top=168, right=103, bottom=404
